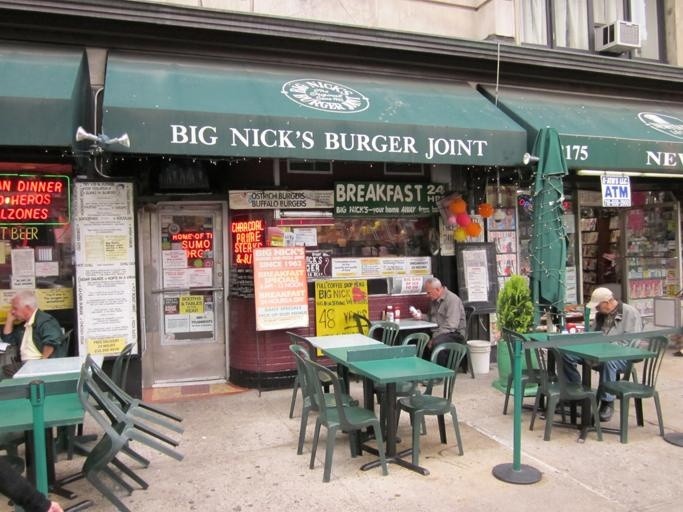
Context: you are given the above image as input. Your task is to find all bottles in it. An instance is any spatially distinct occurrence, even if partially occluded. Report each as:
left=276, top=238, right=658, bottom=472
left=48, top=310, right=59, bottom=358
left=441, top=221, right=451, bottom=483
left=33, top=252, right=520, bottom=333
left=408, top=304, right=420, bottom=317
left=394, top=303, right=401, bottom=318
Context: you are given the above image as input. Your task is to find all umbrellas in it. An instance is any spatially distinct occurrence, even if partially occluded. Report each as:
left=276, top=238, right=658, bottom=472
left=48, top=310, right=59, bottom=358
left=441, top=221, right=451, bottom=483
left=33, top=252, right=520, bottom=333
left=530, top=126, right=569, bottom=334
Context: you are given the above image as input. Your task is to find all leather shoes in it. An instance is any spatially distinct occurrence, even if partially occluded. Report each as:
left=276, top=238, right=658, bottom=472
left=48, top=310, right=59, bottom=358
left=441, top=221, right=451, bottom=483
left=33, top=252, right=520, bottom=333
left=600, top=401, right=615, bottom=422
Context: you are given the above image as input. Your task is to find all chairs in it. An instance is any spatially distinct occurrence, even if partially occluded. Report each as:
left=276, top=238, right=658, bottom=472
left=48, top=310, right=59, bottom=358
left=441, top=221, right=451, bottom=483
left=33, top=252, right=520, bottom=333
left=78, top=342, right=137, bottom=441
left=591, top=335, right=669, bottom=444
left=368, top=321, right=400, bottom=404
left=76, top=365, right=185, bottom=512
left=502, top=326, right=567, bottom=424
left=460, top=305, right=477, bottom=380
left=303, top=357, right=388, bottom=482
left=394, top=342, right=467, bottom=464
left=588, top=361, right=646, bottom=427
left=86, top=354, right=181, bottom=489
left=13, top=358, right=97, bottom=456
left=289, top=343, right=361, bottom=458
left=380, top=332, right=430, bottom=436
left=353, top=312, right=373, bottom=383
left=529, top=345, right=605, bottom=442
left=286, top=330, right=347, bottom=418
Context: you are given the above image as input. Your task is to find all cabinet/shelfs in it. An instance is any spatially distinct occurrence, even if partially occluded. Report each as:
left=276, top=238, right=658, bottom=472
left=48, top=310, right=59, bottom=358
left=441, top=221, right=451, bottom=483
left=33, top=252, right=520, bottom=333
left=581, top=215, right=622, bottom=300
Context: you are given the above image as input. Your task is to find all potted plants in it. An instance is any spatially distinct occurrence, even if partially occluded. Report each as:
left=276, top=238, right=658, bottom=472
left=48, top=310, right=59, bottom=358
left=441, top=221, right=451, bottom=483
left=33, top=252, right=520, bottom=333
left=492, top=275, right=539, bottom=398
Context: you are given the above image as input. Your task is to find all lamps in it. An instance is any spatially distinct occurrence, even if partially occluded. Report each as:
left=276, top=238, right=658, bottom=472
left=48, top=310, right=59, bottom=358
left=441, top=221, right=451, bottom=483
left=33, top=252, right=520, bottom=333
left=75, top=92, right=132, bottom=176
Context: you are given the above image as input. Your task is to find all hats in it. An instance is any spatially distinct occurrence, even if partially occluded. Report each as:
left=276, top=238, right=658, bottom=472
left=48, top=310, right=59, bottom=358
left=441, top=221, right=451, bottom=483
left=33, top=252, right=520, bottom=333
left=585, top=287, right=613, bottom=308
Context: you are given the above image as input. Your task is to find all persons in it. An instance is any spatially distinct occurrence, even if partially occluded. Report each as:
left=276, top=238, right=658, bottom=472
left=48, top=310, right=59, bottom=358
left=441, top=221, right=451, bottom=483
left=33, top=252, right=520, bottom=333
left=0, top=456, right=67, bottom=512
left=561, top=287, right=642, bottom=422
left=1, top=290, right=63, bottom=380
left=409, top=277, right=467, bottom=386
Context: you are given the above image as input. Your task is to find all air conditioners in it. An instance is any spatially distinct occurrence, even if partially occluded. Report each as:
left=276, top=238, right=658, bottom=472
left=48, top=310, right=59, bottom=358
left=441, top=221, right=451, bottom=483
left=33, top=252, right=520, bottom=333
left=594, top=20, right=642, bottom=54
